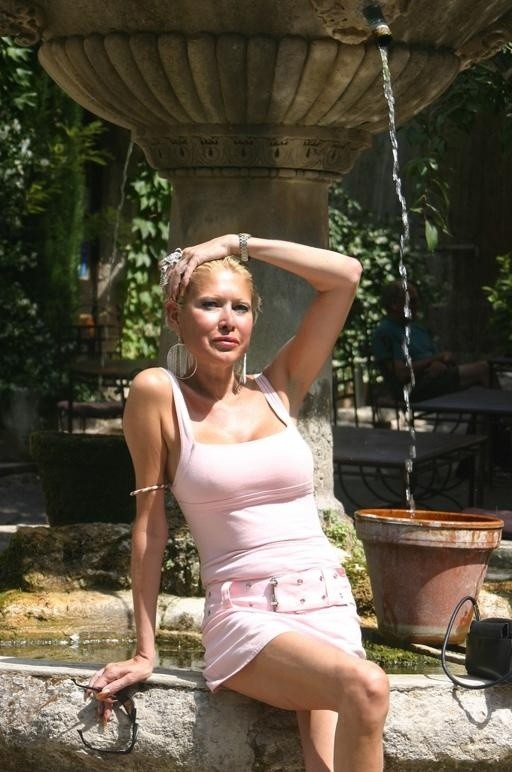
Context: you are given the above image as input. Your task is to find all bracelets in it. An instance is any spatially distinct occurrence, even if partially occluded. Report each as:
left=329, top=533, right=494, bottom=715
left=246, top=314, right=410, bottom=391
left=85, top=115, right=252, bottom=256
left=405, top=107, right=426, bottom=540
left=239, top=232, right=252, bottom=263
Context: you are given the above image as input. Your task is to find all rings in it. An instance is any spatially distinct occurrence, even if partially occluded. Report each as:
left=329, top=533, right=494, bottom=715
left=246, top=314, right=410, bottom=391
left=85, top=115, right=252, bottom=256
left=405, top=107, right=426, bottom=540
left=174, top=247, right=184, bottom=257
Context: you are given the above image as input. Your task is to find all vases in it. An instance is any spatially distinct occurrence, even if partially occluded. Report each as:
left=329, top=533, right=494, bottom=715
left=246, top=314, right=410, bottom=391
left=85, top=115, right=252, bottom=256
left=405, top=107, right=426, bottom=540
left=352, top=509, right=503, bottom=646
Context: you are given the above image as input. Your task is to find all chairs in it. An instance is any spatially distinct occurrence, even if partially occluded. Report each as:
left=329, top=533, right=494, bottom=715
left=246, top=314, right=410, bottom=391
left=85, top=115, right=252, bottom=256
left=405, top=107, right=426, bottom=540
left=360, top=315, right=442, bottom=433
left=57, top=302, right=126, bottom=433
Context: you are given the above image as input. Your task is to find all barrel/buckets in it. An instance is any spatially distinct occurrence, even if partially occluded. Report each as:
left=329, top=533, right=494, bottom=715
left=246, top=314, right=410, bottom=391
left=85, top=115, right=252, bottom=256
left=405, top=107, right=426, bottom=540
left=354, top=506, right=505, bottom=647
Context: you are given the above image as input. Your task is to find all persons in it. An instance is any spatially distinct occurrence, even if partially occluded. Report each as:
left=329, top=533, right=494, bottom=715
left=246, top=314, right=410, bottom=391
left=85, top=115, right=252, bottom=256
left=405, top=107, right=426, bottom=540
left=82, top=231, right=395, bottom=771
left=368, top=278, right=492, bottom=479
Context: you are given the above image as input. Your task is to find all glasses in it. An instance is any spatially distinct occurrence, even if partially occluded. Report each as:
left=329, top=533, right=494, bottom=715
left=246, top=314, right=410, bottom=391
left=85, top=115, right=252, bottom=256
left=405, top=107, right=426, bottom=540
left=68, top=675, right=140, bottom=757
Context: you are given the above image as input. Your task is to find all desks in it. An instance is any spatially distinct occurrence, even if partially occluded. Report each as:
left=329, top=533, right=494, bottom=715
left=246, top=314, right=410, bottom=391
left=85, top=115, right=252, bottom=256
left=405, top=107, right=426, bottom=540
left=330, top=383, right=512, bottom=510
left=58, top=358, right=160, bottom=433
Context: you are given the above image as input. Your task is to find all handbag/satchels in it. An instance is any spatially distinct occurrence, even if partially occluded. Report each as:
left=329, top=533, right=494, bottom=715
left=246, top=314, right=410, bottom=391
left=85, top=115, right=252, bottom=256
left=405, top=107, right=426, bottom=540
left=440, top=595, right=512, bottom=692
